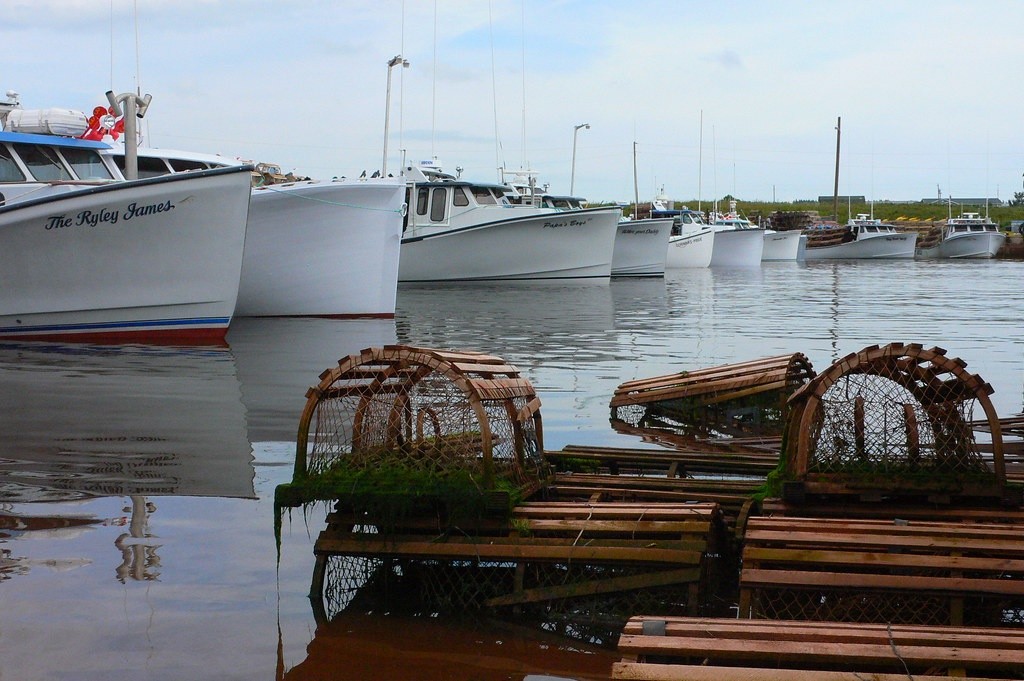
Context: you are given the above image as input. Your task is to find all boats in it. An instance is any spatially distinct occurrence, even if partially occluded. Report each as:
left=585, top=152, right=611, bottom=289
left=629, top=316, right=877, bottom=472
left=399, top=0, right=805, bottom=284
left=0, top=83, right=254, bottom=351
left=800, top=193, right=919, bottom=261
left=913, top=194, right=1009, bottom=261
left=105, top=141, right=408, bottom=322
left=1, top=306, right=411, bottom=586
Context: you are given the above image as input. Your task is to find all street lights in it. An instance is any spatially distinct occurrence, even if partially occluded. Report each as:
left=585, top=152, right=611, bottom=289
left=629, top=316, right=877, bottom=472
left=571, top=122, right=591, bottom=197
left=382, top=55, right=411, bottom=177
